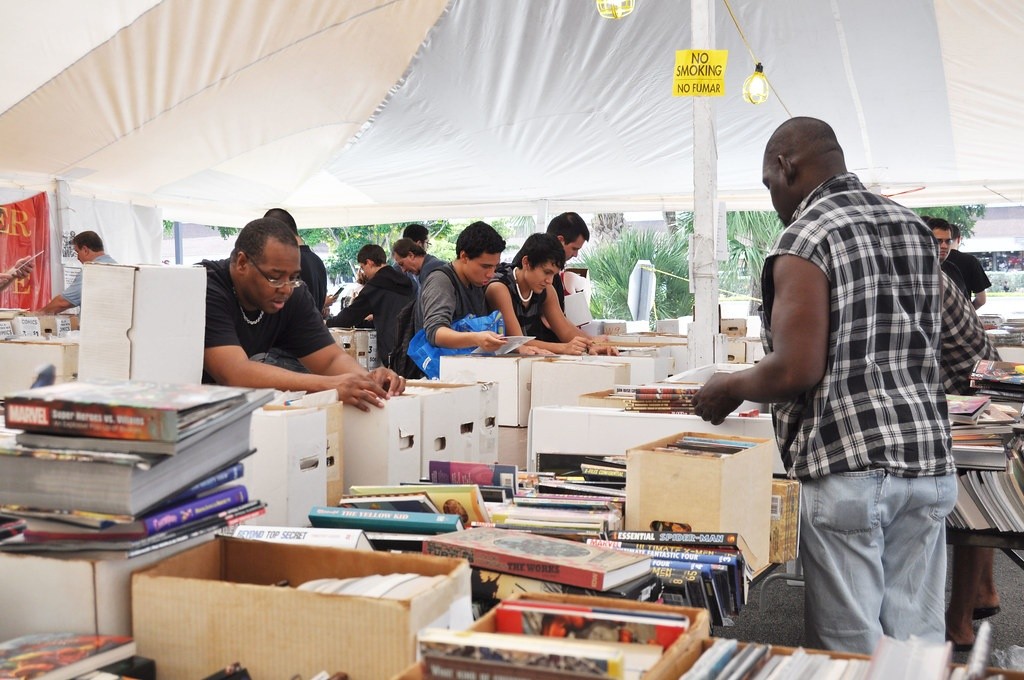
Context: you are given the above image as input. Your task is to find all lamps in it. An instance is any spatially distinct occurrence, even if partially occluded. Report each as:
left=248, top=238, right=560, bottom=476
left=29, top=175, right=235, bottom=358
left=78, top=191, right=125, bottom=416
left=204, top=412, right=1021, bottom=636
left=596, top=0, right=634, bottom=19
left=740, top=62, right=770, bottom=106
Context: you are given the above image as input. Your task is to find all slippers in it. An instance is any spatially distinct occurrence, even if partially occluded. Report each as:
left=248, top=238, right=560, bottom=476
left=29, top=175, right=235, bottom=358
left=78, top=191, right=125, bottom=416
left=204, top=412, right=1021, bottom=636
left=944, top=606, right=1002, bottom=652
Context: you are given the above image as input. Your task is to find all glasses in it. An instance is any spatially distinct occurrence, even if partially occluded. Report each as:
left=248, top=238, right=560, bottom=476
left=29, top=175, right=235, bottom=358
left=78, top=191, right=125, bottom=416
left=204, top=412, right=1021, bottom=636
left=937, top=239, right=954, bottom=245
left=248, top=257, right=304, bottom=289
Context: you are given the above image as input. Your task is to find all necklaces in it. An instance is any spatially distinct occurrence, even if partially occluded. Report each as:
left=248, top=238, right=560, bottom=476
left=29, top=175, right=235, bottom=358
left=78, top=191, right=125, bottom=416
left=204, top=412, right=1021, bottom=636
left=233, top=286, right=264, bottom=325
left=513, top=266, right=533, bottom=302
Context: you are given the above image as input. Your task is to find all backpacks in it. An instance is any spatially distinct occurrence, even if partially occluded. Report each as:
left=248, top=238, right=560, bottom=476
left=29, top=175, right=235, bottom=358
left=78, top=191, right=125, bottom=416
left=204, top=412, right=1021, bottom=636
left=386, top=266, right=464, bottom=380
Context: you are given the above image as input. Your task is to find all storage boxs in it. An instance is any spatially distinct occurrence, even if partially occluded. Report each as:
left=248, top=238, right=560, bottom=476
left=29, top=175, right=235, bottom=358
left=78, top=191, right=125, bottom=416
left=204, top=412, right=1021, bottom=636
left=1, top=259, right=1024, bottom=680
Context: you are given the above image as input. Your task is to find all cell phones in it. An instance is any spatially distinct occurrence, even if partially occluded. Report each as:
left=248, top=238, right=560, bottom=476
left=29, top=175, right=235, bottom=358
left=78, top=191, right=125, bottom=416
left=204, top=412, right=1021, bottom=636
left=333, top=287, right=344, bottom=298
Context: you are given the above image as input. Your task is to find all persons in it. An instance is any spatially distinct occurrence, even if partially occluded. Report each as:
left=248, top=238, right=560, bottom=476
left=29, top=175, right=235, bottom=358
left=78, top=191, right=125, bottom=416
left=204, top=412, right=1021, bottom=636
left=941, top=271, right=999, bottom=651
left=194, top=218, right=406, bottom=412
left=411, top=221, right=556, bottom=382
left=484, top=232, right=620, bottom=358
left=926, top=218, right=970, bottom=302
left=541, top=212, right=611, bottom=343
left=38, top=230, right=117, bottom=316
left=946, top=225, right=991, bottom=311
left=692, top=116, right=958, bottom=653
left=392, top=223, right=429, bottom=278
left=323, top=245, right=417, bottom=368
left=264, top=208, right=328, bottom=312
left=394, top=238, right=445, bottom=283
left=0, top=255, right=36, bottom=292
left=351, top=266, right=375, bottom=329
left=321, top=294, right=339, bottom=317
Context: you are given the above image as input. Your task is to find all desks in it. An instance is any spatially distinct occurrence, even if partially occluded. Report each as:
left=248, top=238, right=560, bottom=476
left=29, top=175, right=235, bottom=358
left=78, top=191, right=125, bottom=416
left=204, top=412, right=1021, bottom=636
left=746, top=525, right=1024, bottom=612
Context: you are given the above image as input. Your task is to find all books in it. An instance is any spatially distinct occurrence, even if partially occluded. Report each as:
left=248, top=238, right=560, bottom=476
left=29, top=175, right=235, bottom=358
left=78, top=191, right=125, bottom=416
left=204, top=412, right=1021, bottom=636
left=0, top=305, right=1024, bottom=680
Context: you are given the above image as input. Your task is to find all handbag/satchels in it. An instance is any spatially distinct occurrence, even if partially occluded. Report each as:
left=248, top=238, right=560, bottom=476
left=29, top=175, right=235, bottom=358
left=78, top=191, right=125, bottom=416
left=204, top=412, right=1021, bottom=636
left=407, top=309, right=505, bottom=380
